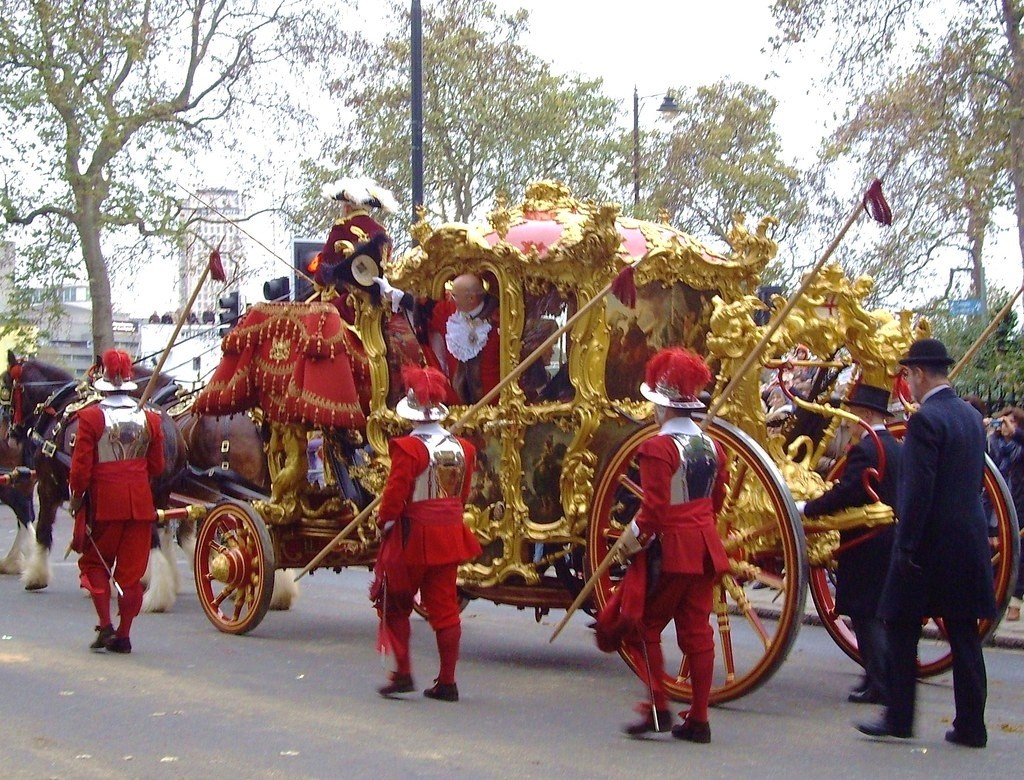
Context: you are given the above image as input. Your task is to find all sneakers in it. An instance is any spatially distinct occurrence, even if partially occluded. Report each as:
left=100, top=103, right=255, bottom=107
left=90, top=624, right=132, bottom=653
left=424, top=678, right=459, bottom=702
left=672, top=710, right=712, bottom=744
left=627, top=710, right=672, bottom=735
left=378, top=672, right=415, bottom=694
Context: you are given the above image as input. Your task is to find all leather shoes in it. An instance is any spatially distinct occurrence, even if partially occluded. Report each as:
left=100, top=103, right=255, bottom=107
left=847, top=688, right=876, bottom=702
left=855, top=721, right=912, bottom=739
left=944, top=728, right=987, bottom=749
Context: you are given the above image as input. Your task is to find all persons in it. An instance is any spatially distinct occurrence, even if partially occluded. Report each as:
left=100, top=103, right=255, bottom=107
left=373, top=365, right=479, bottom=702
left=521, top=310, right=560, bottom=401
left=595, top=349, right=718, bottom=739
left=372, top=272, right=502, bottom=411
left=792, top=384, right=906, bottom=705
left=962, top=393, right=1024, bottom=622
left=852, top=339, right=991, bottom=748
left=69, top=349, right=164, bottom=654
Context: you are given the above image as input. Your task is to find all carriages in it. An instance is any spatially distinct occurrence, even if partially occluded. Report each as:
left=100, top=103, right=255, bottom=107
left=0, top=179, right=1020, bottom=705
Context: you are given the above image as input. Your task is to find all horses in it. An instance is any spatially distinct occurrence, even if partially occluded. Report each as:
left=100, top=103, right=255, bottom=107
left=91, top=352, right=299, bottom=615
left=1, top=347, right=189, bottom=614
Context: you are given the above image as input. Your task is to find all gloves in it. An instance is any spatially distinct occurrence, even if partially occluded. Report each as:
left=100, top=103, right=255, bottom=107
left=372, top=275, right=394, bottom=299
left=795, top=500, right=808, bottom=514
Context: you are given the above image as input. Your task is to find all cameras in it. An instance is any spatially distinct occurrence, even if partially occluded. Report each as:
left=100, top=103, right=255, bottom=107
left=989, top=420, right=1002, bottom=429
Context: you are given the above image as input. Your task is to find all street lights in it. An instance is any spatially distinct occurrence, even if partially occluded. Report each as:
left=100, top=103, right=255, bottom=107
left=634, top=88, right=680, bottom=202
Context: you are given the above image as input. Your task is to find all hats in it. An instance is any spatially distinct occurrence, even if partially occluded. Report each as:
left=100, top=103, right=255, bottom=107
left=898, top=339, right=956, bottom=366
left=320, top=175, right=399, bottom=215
left=95, top=349, right=139, bottom=392
left=842, top=383, right=895, bottom=418
left=395, top=367, right=450, bottom=423
left=640, top=348, right=708, bottom=410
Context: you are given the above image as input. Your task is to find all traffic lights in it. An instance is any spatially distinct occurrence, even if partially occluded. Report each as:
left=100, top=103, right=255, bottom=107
left=263, top=276, right=289, bottom=302
left=219, top=291, right=239, bottom=339
left=290, top=240, right=326, bottom=303
